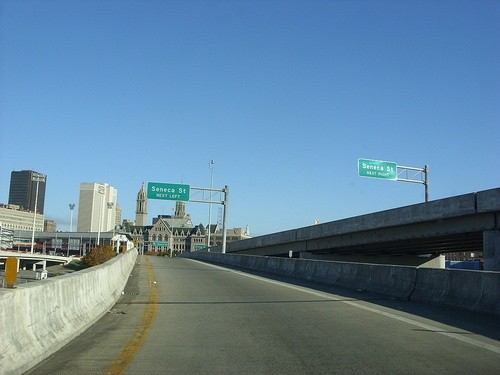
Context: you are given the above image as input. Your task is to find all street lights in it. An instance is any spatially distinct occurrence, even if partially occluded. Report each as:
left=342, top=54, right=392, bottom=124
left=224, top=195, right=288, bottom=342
left=31, top=172, right=46, bottom=255
left=208, top=159, right=215, bottom=250
left=106, top=202, right=114, bottom=232
left=69, top=203, right=75, bottom=232
